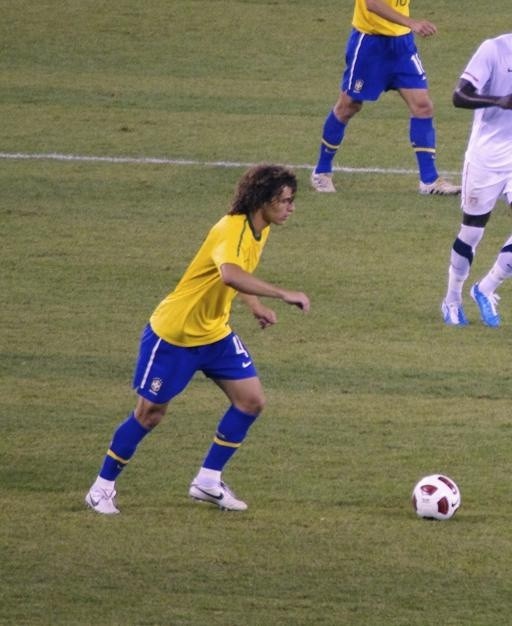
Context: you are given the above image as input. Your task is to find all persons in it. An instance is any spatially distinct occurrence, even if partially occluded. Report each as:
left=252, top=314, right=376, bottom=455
left=441, top=32, right=512, bottom=328
left=310, top=0, right=461, bottom=195
left=85, top=163, right=309, bottom=514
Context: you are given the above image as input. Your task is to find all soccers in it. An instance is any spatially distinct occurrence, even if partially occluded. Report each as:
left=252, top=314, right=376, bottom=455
left=411, top=474, right=461, bottom=521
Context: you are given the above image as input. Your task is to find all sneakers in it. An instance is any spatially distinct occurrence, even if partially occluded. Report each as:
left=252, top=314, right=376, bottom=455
left=310, top=167, right=337, bottom=194
left=84, top=489, right=120, bottom=515
left=468, top=281, right=502, bottom=328
left=418, top=177, right=463, bottom=197
left=440, top=297, right=470, bottom=328
left=188, top=479, right=249, bottom=513
